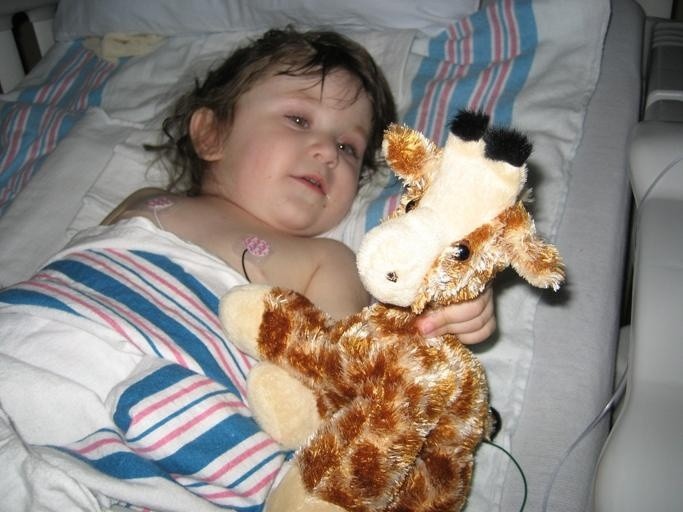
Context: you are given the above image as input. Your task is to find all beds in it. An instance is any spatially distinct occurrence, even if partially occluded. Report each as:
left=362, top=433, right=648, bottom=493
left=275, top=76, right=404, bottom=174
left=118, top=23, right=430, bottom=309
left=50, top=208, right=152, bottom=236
left=0, top=1, right=647, bottom=511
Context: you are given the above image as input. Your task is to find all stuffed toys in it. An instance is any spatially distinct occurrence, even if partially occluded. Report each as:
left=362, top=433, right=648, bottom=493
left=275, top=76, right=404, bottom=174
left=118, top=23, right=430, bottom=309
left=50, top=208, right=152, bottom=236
left=218, top=109, right=565, bottom=512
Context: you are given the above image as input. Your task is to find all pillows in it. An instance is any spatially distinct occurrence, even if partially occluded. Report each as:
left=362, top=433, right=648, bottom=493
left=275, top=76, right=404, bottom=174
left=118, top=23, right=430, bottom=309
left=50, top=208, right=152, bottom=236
left=49, top=2, right=482, bottom=41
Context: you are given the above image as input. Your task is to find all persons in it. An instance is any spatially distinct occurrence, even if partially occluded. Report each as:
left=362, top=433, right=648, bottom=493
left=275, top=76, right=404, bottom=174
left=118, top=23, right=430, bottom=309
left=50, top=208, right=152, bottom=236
left=97, top=24, right=497, bottom=346
